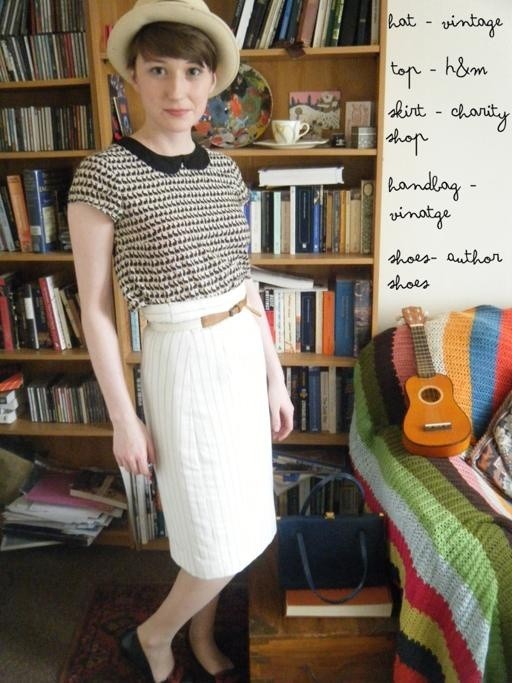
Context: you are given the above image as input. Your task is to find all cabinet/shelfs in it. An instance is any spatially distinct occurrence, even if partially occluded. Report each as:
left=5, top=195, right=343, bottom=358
left=95, top=0, right=385, bottom=551
left=2, top=0, right=140, bottom=548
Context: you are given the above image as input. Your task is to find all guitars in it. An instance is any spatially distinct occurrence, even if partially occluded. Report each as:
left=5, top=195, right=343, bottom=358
left=395, top=306, right=472, bottom=458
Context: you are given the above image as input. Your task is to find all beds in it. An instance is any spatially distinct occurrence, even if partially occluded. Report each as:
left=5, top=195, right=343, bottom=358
left=350, top=306, right=512, bottom=681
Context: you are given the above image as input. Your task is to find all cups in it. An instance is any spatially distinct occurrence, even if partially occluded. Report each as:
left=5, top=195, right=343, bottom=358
left=272, top=120, right=309, bottom=144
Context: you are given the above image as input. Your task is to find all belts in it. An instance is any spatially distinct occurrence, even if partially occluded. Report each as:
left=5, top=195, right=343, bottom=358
left=147, top=293, right=263, bottom=332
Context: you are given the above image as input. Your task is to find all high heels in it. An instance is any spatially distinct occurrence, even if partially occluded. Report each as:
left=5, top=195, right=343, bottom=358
left=114, top=625, right=185, bottom=683
left=179, top=634, right=242, bottom=682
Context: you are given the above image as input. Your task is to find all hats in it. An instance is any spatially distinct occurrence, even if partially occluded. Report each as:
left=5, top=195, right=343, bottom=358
left=276, top=470, right=389, bottom=604
left=105, top=0, right=242, bottom=99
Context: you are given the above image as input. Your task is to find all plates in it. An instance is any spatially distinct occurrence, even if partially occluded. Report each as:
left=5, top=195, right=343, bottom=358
left=253, top=139, right=329, bottom=150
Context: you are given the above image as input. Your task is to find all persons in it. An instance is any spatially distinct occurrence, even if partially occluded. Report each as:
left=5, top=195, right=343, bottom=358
left=67, top=1, right=295, bottom=683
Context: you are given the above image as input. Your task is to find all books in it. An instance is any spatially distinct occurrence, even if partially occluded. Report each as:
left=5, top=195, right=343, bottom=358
left=251, top=266, right=373, bottom=355
left=231, top=0, right=381, bottom=49
left=0, top=169, right=71, bottom=252
left=279, top=363, right=356, bottom=434
left=1, top=265, right=82, bottom=351
left=272, top=454, right=345, bottom=517
left=0, top=0, right=89, bottom=82
left=240, top=164, right=374, bottom=258
left=0, top=449, right=167, bottom=551
left=0, top=373, right=111, bottom=426
left=0, top=105, right=95, bottom=151
left=128, top=303, right=148, bottom=351
